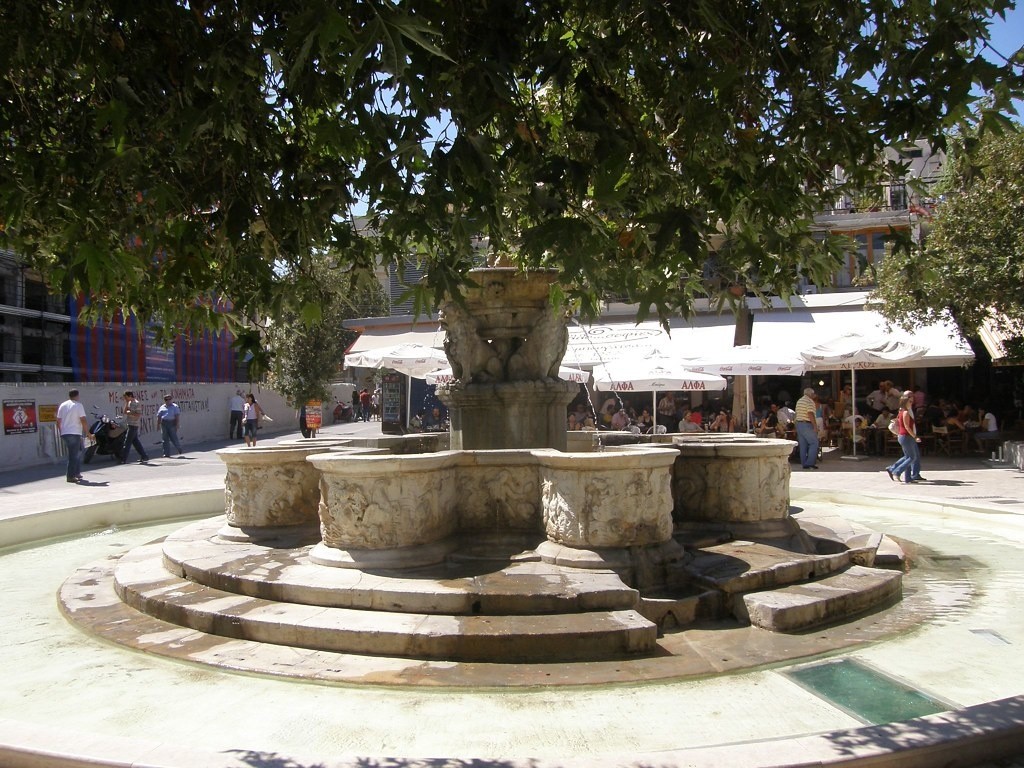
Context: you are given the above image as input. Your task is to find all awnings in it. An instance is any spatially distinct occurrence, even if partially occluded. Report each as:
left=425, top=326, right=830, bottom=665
left=347, top=305, right=974, bottom=373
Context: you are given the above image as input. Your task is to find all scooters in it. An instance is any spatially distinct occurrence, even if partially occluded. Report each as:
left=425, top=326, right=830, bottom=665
left=332, top=395, right=353, bottom=425
left=82, top=405, right=128, bottom=464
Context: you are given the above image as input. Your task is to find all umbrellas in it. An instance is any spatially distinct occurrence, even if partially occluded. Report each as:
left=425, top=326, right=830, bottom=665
left=346, top=344, right=451, bottom=428
left=685, top=345, right=807, bottom=433
left=800, top=332, right=930, bottom=455
left=598, top=347, right=727, bottom=434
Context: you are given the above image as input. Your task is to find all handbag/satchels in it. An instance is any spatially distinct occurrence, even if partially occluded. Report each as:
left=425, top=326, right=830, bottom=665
left=241, top=418, right=247, bottom=426
left=888, top=412, right=901, bottom=436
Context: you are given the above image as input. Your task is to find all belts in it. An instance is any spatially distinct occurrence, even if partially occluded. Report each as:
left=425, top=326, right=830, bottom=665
left=799, top=421, right=811, bottom=423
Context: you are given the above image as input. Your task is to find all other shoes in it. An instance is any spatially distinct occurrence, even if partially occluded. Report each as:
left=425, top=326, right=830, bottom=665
left=121, top=459, right=126, bottom=464
left=893, top=472, right=901, bottom=482
left=163, top=454, right=170, bottom=457
left=67, top=477, right=77, bottom=482
left=886, top=467, right=895, bottom=481
left=914, top=477, right=926, bottom=480
left=807, top=464, right=818, bottom=468
left=975, top=448, right=985, bottom=453
left=136, top=457, right=149, bottom=462
left=803, top=466, right=809, bottom=469
left=75, top=473, right=82, bottom=478
left=908, top=480, right=918, bottom=483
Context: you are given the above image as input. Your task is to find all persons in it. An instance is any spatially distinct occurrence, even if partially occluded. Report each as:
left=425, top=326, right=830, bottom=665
left=157, top=395, right=183, bottom=457
left=567, top=380, right=1024, bottom=483
left=56, top=388, right=96, bottom=482
left=352, top=388, right=382, bottom=422
left=230, top=389, right=273, bottom=447
left=296, top=406, right=312, bottom=439
left=409, top=408, right=428, bottom=433
left=795, top=388, right=818, bottom=469
left=118, top=390, right=149, bottom=462
left=424, top=409, right=446, bottom=432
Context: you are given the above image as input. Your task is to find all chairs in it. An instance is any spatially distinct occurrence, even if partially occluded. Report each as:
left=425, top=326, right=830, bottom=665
left=982, top=420, right=1005, bottom=460
left=930, top=413, right=964, bottom=458
left=882, top=430, right=904, bottom=458
left=842, top=422, right=864, bottom=452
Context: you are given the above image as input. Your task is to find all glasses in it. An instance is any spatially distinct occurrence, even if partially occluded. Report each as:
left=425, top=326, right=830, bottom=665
left=165, top=398, right=172, bottom=401
